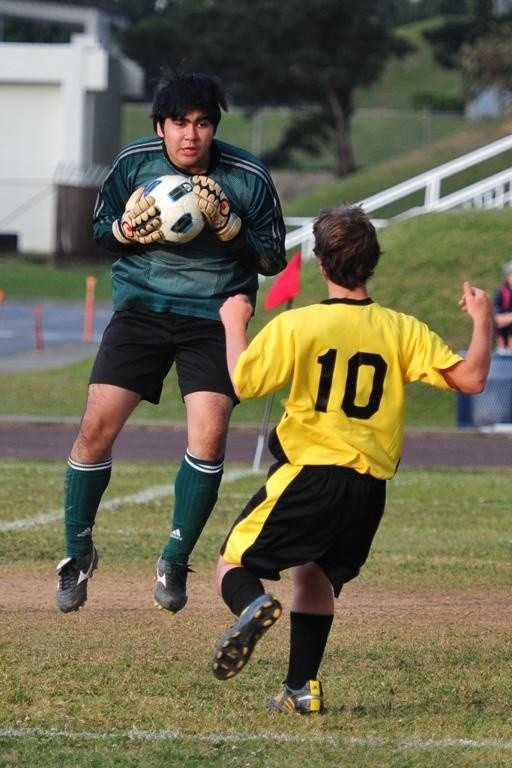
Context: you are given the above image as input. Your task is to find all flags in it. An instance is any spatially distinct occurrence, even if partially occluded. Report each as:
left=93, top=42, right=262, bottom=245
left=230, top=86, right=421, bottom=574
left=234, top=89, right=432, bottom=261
left=265, top=251, right=300, bottom=312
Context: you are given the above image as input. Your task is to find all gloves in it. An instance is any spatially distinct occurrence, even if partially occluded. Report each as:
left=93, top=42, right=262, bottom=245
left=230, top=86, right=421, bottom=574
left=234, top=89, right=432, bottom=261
left=188, top=173, right=242, bottom=243
left=110, top=185, right=164, bottom=247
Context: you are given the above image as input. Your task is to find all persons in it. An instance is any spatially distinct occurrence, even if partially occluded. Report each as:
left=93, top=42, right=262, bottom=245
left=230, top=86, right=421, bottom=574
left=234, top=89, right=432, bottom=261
left=492, top=272, right=512, bottom=349
left=212, top=200, right=494, bottom=714
left=54, top=72, right=286, bottom=612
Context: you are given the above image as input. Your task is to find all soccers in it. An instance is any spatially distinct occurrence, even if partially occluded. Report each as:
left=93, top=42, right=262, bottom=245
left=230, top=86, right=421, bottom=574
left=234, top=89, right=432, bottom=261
left=140, top=175, right=204, bottom=245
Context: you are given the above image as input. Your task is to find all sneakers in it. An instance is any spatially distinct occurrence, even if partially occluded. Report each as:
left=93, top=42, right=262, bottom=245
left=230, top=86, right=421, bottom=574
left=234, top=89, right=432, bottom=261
left=55, top=544, right=99, bottom=615
left=263, top=677, right=326, bottom=716
left=150, top=547, right=197, bottom=614
left=209, top=593, right=284, bottom=681
